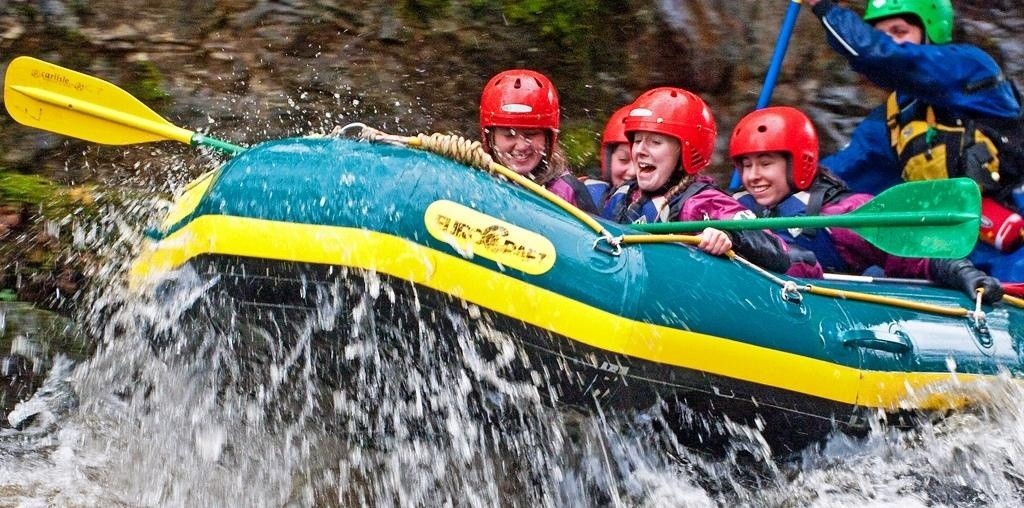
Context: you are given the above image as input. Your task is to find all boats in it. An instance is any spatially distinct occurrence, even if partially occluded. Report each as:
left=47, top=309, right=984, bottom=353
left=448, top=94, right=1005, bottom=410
left=129, top=138, right=1024, bottom=495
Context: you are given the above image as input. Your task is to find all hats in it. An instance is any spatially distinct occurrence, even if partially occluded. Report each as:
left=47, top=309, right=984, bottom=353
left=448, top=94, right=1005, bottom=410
left=864, top=1, right=956, bottom=47
left=626, top=88, right=718, bottom=177
left=733, top=106, right=822, bottom=190
left=479, top=69, right=559, bottom=162
left=600, top=105, right=630, bottom=176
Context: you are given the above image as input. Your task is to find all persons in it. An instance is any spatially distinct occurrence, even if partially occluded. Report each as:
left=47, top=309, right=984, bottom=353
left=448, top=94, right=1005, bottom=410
left=596, top=85, right=791, bottom=274
left=479, top=68, right=600, bottom=218
left=803, top=0, right=1024, bottom=285
left=722, top=105, right=1006, bottom=304
left=577, top=104, right=637, bottom=213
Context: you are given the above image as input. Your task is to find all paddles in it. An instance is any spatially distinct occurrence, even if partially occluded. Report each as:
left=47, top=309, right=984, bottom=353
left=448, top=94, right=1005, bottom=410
left=622, top=178, right=982, bottom=260
left=2, top=55, right=249, bottom=155
